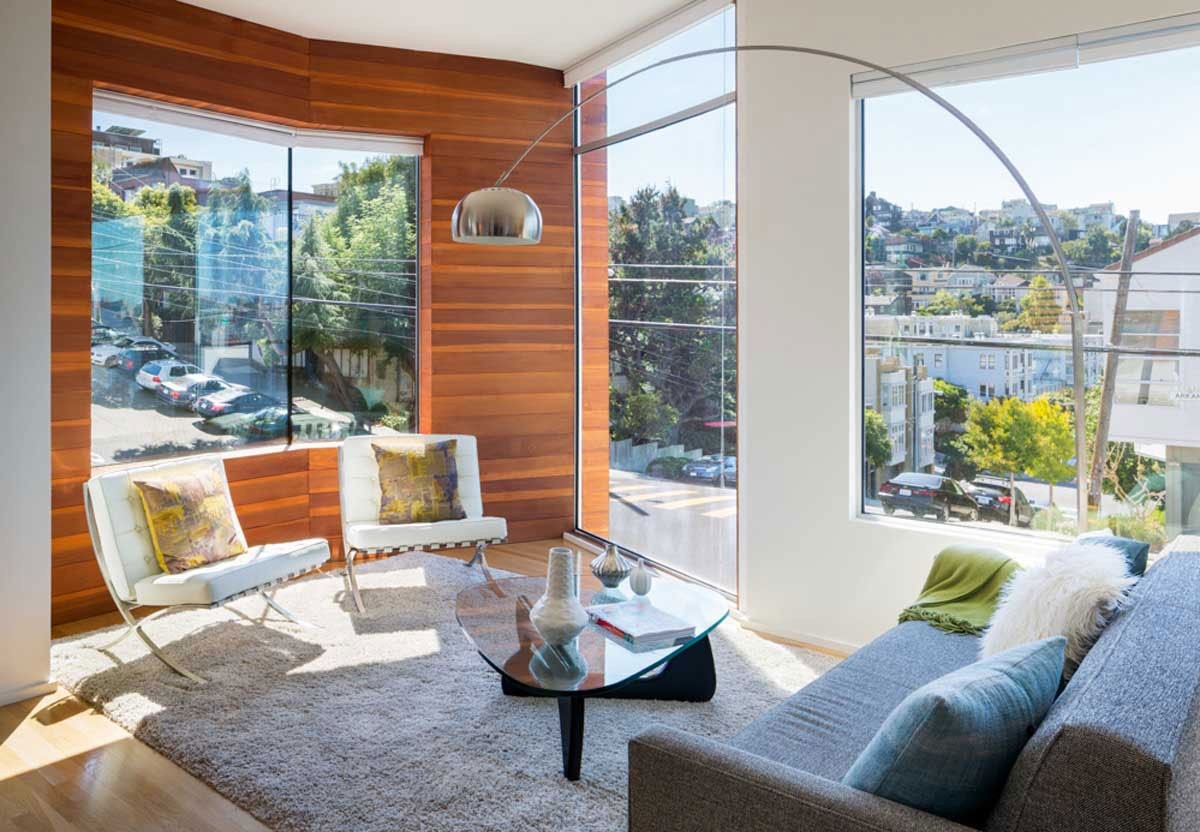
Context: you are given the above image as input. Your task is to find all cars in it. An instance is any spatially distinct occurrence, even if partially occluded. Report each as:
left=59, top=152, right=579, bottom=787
left=137, top=358, right=207, bottom=392
left=198, top=385, right=278, bottom=417
left=156, top=372, right=250, bottom=414
left=91, top=335, right=177, bottom=367
left=117, top=348, right=182, bottom=377
left=876, top=470, right=978, bottom=521
left=680, top=454, right=738, bottom=486
left=243, top=405, right=307, bottom=440
left=1025, top=498, right=1079, bottom=523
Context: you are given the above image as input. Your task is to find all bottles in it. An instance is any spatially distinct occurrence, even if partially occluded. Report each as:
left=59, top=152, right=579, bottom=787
left=590, top=544, right=632, bottom=587
left=530, top=546, right=590, bottom=645
left=630, top=558, right=652, bottom=595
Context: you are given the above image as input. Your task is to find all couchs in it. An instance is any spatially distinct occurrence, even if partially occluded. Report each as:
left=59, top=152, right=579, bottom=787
left=627, top=527, right=1200, bottom=832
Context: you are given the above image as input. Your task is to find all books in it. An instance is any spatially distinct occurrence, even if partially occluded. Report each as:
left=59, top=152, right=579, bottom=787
left=586, top=597, right=697, bottom=644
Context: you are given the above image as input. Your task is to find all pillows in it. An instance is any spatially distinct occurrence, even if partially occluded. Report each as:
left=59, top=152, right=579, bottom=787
left=840, top=635, right=1072, bottom=825
left=1075, top=536, right=1151, bottom=578
left=129, top=464, right=248, bottom=575
left=369, top=438, right=468, bottom=525
left=975, top=538, right=1142, bottom=691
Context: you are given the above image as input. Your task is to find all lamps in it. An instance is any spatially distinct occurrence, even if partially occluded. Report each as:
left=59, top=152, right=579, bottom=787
left=449, top=44, right=1089, bottom=536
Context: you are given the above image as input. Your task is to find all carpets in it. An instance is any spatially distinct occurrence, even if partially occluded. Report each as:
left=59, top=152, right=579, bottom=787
left=49, top=552, right=844, bottom=832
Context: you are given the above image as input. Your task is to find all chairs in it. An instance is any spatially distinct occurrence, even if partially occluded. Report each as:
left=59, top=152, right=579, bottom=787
left=82, top=451, right=332, bottom=687
left=336, top=432, right=508, bottom=615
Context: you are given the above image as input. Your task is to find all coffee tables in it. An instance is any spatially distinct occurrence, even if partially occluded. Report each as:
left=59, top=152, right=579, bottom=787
left=454, top=567, right=733, bottom=781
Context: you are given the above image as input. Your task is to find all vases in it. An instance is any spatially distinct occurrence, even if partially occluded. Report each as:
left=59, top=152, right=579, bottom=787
left=629, top=557, right=652, bottom=596
left=589, top=543, right=631, bottom=588
left=528, top=547, right=589, bottom=647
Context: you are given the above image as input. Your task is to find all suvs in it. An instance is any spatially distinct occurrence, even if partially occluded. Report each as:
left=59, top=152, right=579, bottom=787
left=964, top=473, right=1032, bottom=526
